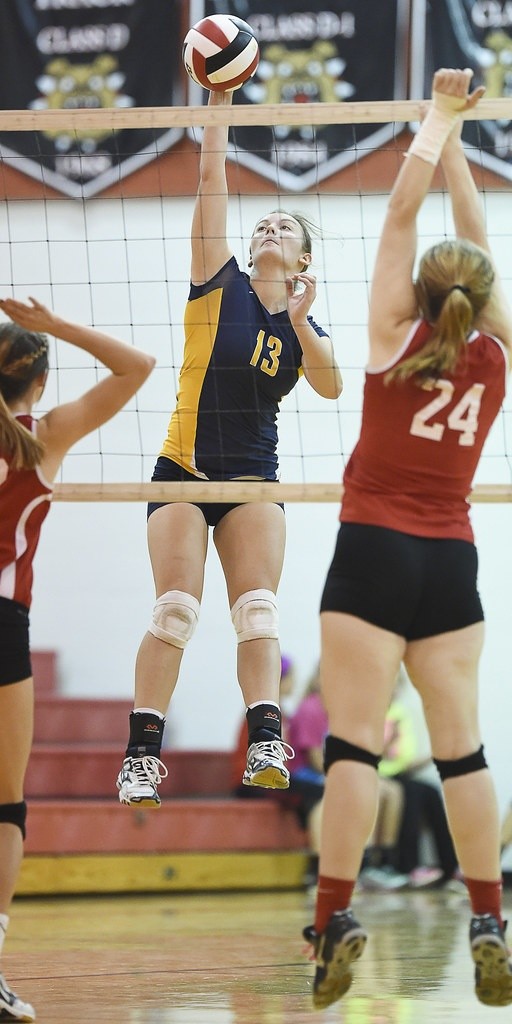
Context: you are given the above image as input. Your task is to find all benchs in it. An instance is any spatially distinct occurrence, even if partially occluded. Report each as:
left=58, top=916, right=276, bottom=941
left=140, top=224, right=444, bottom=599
left=10, top=651, right=313, bottom=900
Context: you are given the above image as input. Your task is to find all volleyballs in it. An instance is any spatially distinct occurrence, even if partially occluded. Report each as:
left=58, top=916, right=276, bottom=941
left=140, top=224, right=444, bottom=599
left=181, top=14, right=261, bottom=91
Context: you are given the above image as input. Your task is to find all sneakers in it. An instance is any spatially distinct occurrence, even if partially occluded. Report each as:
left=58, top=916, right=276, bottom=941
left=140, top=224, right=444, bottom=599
left=470, top=914, right=512, bottom=1008
left=309, top=907, right=368, bottom=1012
left=116, top=746, right=169, bottom=809
left=242, top=727, right=295, bottom=791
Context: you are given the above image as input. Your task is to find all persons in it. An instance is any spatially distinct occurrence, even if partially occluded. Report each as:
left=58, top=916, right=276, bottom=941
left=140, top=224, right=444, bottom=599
left=0, top=296, right=160, bottom=1024
left=115, top=90, right=345, bottom=809
left=235, top=651, right=511, bottom=904
left=301, top=65, right=511, bottom=1008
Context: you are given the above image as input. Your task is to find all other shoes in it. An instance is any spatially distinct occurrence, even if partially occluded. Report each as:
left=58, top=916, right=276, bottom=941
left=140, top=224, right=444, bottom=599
left=409, top=867, right=444, bottom=890
left=364, top=863, right=410, bottom=890
left=0, top=977, right=35, bottom=1022
